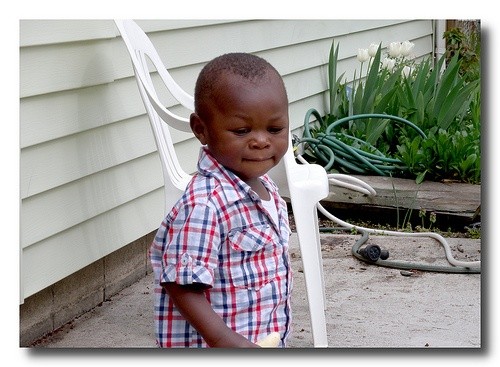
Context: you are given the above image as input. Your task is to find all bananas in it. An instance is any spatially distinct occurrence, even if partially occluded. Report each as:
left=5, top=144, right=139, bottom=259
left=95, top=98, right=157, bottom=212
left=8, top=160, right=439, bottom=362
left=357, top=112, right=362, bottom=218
left=255, top=332, right=281, bottom=347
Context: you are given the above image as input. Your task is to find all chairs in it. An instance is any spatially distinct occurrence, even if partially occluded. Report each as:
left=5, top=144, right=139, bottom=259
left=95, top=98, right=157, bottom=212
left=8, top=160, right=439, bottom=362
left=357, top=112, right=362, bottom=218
left=114, top=19, right=330, bottom=347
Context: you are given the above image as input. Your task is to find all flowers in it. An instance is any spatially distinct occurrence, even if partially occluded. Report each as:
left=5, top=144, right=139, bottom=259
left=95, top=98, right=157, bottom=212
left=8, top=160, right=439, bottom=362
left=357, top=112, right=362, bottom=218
left=357, top=40, right=414, bottom=83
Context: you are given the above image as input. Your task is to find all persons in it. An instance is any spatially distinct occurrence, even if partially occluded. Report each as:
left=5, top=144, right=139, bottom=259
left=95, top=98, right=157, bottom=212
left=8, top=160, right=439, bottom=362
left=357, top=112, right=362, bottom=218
left=150, top=52, right=293, bottom=347
